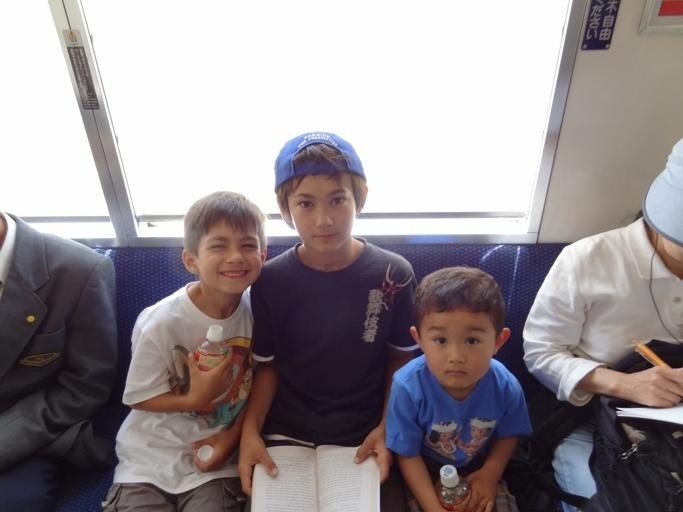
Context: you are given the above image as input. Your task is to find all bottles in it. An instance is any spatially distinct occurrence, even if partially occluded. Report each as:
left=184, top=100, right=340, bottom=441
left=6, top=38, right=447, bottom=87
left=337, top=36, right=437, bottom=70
left=196, top=324, right=228, bottom=371
left=435, top=464, right=471, bottom=511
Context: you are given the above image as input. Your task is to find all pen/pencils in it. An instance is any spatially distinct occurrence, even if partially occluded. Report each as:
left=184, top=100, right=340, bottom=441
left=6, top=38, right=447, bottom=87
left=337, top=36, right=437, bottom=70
left=631, top=338, right=672, bottom=370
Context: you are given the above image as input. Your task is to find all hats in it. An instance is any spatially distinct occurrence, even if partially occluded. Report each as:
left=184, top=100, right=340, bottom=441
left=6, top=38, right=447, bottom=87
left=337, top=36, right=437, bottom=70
left=640, top=136, right=683, bottom=249
left=273, top=131, right=366, bottom=192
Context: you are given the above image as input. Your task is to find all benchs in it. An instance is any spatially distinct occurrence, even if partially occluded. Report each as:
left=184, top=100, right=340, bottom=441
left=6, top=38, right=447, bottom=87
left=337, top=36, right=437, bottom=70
left=47, top=243, right=570, bottom=511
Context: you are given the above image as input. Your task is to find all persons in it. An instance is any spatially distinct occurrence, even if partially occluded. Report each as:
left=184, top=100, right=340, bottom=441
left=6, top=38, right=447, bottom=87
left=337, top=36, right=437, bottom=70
left=385, top=264, right=533, bottom=512
left=0, top=212, right=123, bottom=512
left=105, top=191, right=270, bottom=512
left=523, top=139, right=683, bottom=512
left=237, top=133, right=421, bottom=511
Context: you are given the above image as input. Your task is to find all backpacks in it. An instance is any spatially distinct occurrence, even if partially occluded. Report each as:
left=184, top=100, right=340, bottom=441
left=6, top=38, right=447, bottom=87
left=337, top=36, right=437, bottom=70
left=524, top=338, right=683, bottom=512
